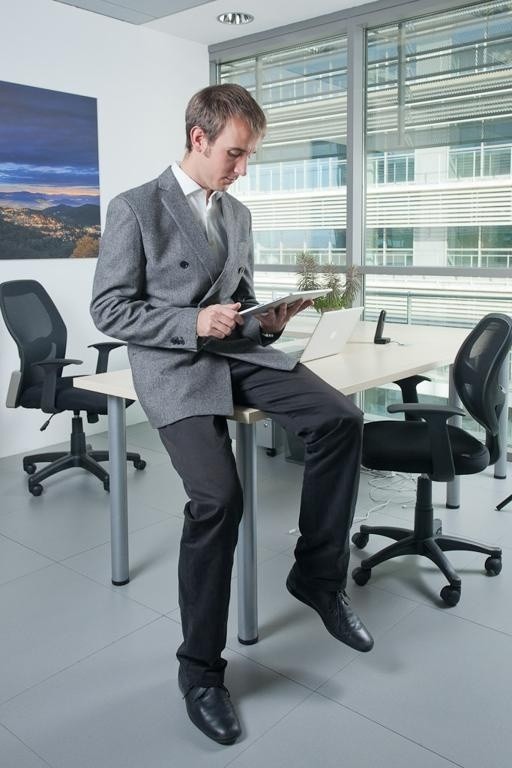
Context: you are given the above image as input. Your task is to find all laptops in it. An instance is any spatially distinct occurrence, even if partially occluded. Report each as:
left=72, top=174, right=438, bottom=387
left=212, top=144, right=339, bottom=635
left=279, top=306, right=364, bottom=364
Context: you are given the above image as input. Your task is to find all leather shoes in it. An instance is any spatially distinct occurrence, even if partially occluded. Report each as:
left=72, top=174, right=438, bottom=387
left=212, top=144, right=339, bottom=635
left=286, top=561, right=374, bottom=653
left=178, top=668, right=241, bottom=744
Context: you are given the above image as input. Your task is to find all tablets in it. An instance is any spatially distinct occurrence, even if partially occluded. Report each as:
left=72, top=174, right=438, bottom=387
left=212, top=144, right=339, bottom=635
left=237, top=288, right=333, bottom=316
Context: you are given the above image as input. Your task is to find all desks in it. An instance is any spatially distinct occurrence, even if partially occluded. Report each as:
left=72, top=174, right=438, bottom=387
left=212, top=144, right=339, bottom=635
left=71, top=306, right=507, bottom=647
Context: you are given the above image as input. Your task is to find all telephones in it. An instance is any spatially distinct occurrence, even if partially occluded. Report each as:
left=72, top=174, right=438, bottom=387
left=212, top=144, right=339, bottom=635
left=374, top=310, right=390, bottom=344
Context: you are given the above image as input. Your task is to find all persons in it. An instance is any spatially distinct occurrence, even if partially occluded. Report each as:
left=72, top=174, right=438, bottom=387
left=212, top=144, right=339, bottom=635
left=90, top=84, right=375, bottom=745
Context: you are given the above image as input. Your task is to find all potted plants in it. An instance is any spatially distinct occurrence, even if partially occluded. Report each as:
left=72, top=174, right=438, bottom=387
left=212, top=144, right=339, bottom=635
left=296, top=250, right=363, bottom=313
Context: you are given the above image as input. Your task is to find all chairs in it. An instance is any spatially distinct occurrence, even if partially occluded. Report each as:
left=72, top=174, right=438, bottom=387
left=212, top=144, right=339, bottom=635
left=351, top=312, right=510, bottom=612
left=1, top=278, right=147, bottom=498
